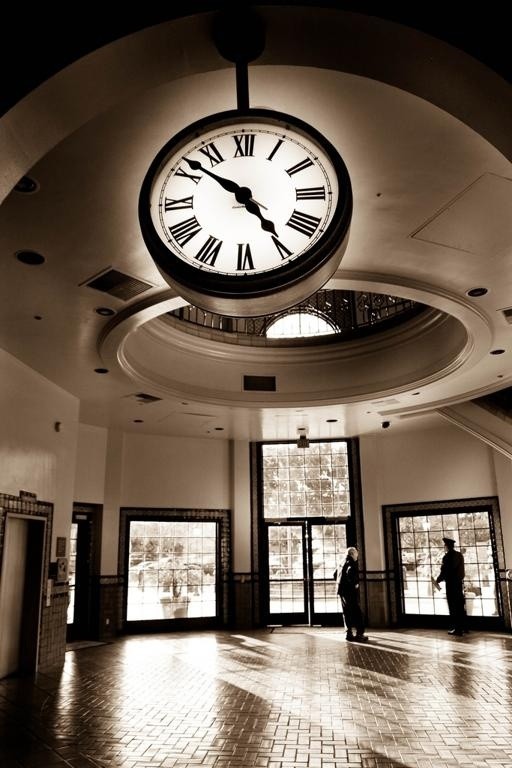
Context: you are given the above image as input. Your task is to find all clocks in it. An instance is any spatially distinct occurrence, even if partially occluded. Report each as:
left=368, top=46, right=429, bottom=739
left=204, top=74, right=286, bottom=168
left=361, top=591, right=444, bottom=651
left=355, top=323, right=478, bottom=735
left=132, top=104, right=357, bottom=318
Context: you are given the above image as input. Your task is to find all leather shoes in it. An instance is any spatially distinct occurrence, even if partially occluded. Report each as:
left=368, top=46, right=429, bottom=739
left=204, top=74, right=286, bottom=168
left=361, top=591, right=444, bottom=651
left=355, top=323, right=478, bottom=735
left=346, top=631, right=368, bottom=642
left=448, top=628, right=469, bottom=636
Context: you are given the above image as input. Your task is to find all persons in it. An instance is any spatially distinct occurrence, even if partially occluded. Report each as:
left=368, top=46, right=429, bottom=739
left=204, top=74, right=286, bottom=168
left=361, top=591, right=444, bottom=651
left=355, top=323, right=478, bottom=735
left=436, top=538, right=469, bottom=636
left=333, top=547, right=368, bottom=642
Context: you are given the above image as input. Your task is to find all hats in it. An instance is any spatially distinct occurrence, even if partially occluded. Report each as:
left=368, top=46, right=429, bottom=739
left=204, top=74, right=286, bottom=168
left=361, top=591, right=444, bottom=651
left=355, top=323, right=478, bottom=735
left=443, top=538, right=456, bottom=544
